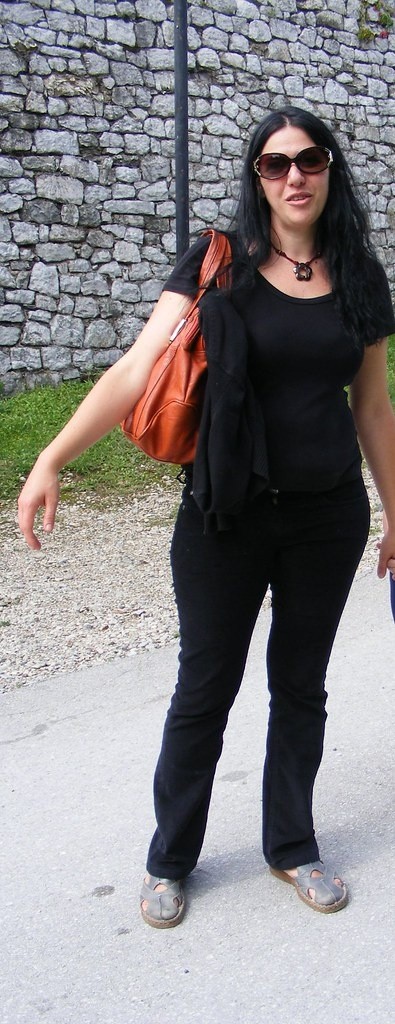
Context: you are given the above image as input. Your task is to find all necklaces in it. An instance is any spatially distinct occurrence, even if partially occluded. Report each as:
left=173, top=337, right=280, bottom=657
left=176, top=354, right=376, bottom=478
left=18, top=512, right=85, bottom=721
left=264, top=234, right=323, bottom=282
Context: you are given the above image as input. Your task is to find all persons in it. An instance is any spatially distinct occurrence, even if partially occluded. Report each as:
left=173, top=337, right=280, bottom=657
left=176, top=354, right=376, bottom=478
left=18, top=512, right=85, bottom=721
left=16, top=108, right=395, bottom=930
left=377, top=503, right=395, bottom=625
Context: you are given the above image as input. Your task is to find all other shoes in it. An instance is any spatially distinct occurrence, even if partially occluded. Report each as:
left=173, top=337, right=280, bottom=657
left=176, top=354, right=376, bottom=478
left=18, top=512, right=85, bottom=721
left=139, top=875, right=186, bottom=929
left=268, top=862, right=348, bottom=913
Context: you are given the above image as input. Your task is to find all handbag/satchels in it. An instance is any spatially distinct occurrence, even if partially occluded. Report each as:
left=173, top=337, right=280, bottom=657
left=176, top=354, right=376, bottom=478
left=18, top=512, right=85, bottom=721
left=124, top=228, right=230, bottom=462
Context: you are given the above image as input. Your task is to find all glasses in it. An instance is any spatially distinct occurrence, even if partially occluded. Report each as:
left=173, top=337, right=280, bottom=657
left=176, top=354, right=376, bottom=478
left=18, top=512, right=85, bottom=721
left=253, top=146, right=335, bottom=181
